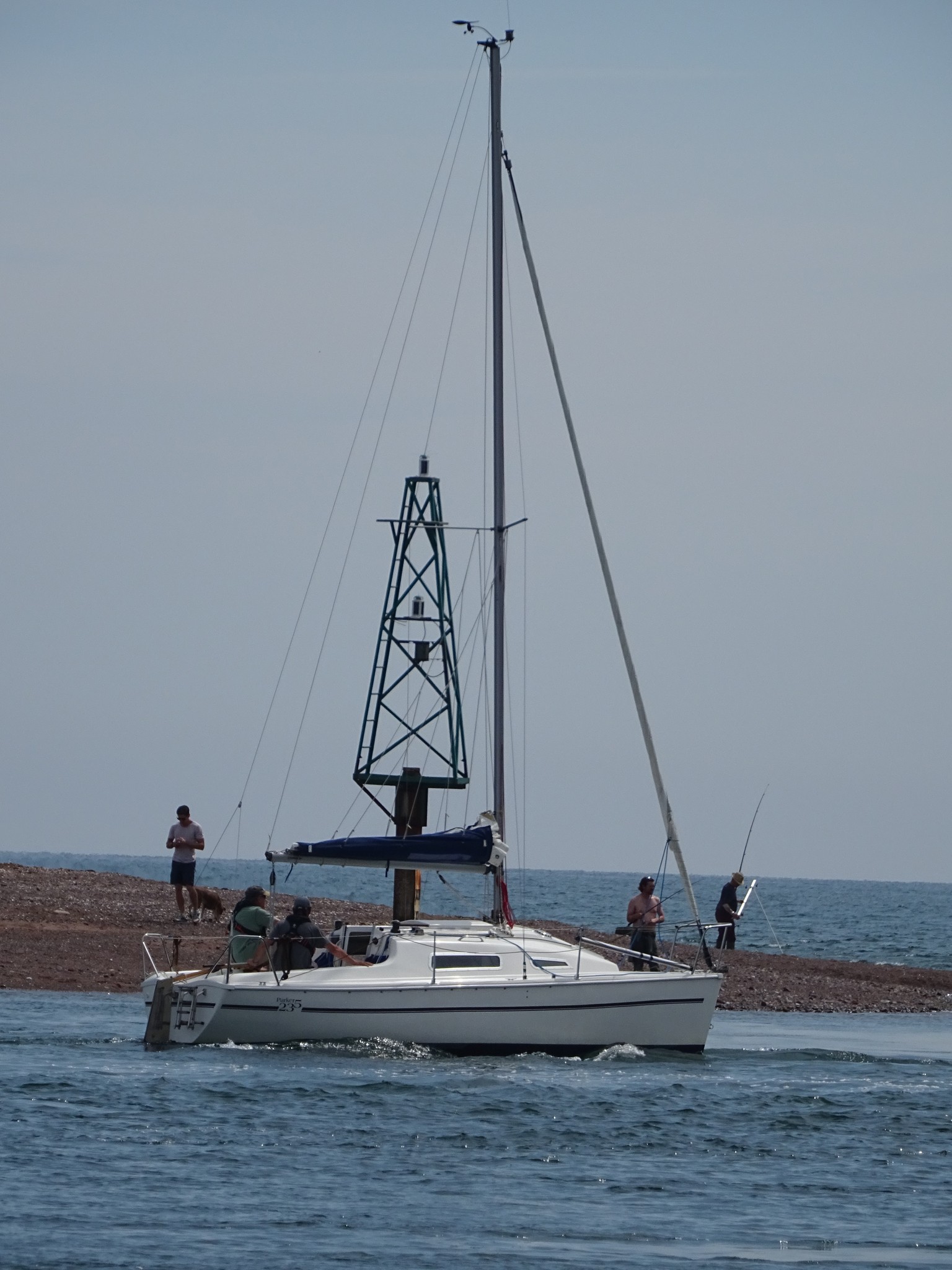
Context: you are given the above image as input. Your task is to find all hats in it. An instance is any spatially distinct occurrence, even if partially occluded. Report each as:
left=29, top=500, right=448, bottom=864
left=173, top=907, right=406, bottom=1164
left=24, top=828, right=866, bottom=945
left=732, top=871, right=745, bottom=885
left=294, top=896, right=312, bottom=909
left=245, top=885, right=270, bottom=897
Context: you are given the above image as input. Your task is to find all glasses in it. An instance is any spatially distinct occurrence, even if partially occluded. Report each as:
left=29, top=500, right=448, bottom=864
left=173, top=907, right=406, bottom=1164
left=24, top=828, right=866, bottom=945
left=646, top=876, right=651, bottom=880
left=176, top=815, right=187, bottom=821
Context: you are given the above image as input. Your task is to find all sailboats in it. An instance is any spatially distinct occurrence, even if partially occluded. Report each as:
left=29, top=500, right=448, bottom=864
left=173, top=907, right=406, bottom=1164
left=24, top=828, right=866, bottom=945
left=138, top=20, right=733, bottom=1060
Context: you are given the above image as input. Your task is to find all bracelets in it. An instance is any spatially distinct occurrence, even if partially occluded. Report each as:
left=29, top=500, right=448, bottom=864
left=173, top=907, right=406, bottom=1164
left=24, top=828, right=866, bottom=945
left=731, top=911, right=734, bottom=915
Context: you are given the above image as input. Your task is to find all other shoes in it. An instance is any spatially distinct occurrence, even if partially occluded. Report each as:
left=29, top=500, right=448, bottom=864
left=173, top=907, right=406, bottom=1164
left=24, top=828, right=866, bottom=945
left=192, top=909, right=201, bottom=923
left=172, top=916, right=188, bottom=923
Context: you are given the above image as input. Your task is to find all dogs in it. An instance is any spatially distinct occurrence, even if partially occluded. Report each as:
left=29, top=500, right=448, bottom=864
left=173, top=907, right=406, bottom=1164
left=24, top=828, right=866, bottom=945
left=188, top=886, right=227, bottom=925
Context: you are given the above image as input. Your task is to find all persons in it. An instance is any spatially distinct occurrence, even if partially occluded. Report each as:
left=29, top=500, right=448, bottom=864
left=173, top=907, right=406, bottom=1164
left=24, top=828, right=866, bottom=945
left=165, top=805, right=205, bottom=924
left=229, top=885, right=280, bottom=968
left=247, top=895, right=374, bottom=972
left=715, top=872, right=744, bottom=950
left=626, top=876, right=665, bottom=972
